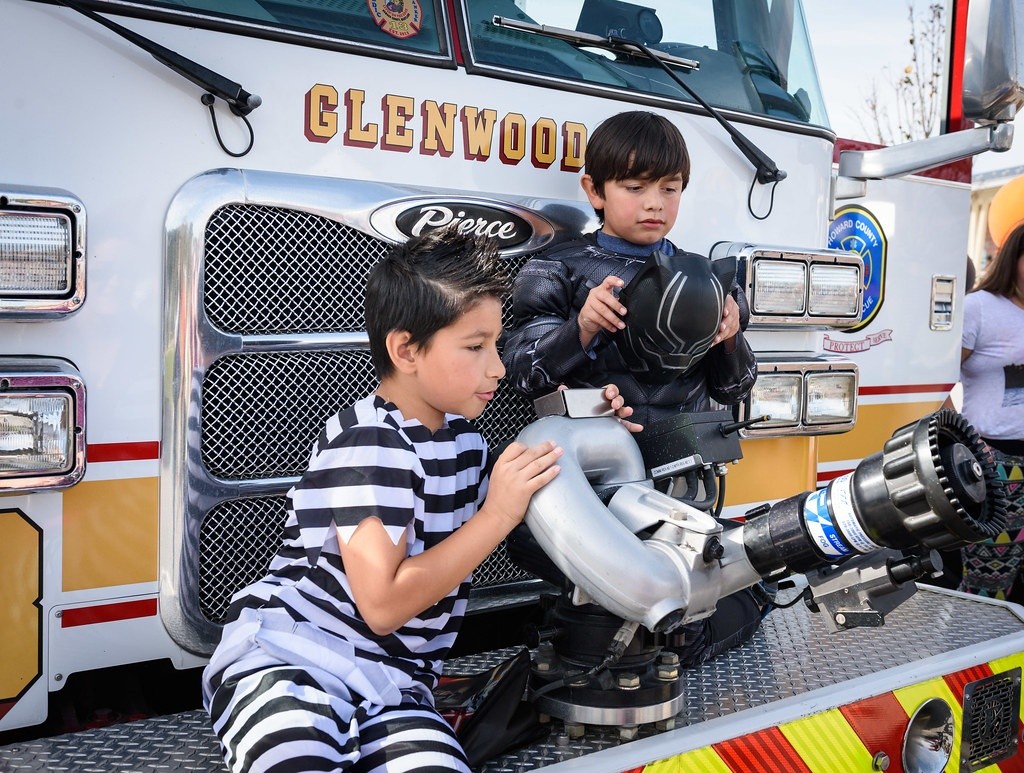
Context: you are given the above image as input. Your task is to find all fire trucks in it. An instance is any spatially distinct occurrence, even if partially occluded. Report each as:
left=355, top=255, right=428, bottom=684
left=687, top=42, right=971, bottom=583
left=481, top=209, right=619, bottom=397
left=0, top=0, right=1024, bottom=773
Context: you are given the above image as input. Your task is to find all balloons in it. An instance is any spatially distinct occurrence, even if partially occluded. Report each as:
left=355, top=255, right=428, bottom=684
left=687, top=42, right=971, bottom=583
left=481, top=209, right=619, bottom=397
left=987, top=176, right=1024, bottom=247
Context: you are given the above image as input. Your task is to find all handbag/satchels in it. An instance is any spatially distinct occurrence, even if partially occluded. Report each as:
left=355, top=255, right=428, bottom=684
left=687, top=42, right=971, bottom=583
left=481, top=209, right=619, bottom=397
left=432, top=646, right=550, bottom=764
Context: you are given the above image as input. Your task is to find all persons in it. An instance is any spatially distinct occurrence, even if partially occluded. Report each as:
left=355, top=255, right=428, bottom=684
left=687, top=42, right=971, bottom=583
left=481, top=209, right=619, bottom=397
left=508, top=111, right=777, bottom=667
left=202, top=230, right=643, bottom=773
left=956, top=224, right=1024, bottom=600
